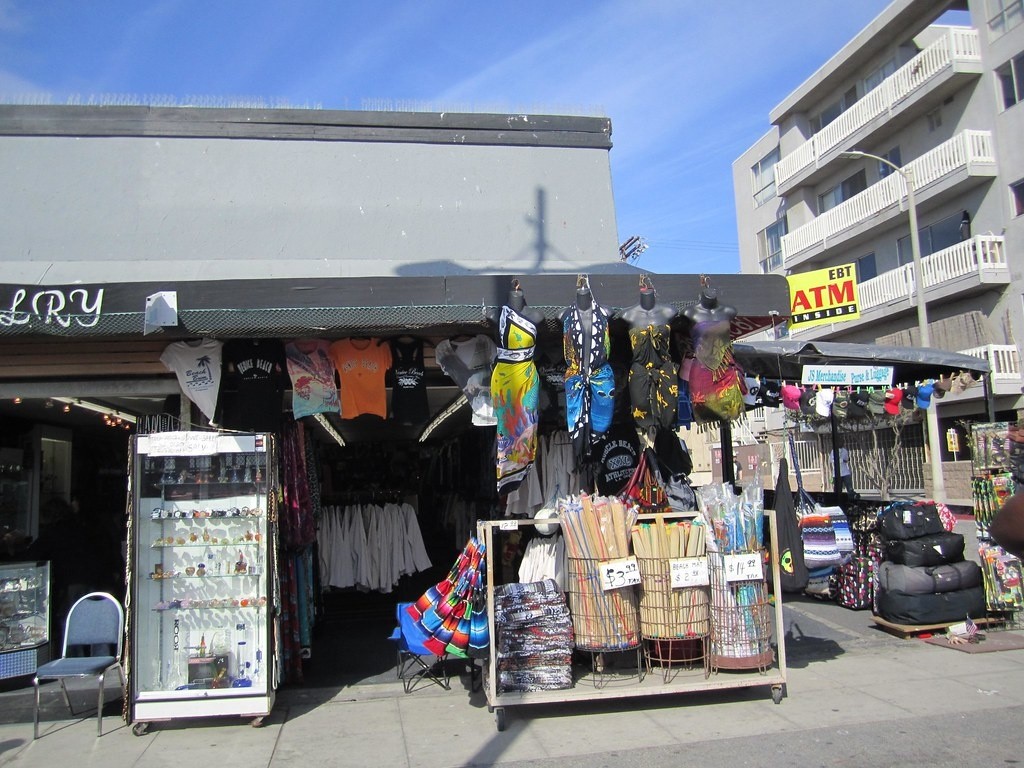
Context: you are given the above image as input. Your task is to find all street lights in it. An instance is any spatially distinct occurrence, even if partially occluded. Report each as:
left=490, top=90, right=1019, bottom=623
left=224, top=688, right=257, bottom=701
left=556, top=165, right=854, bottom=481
left=840, top=148, right=947, bottom=512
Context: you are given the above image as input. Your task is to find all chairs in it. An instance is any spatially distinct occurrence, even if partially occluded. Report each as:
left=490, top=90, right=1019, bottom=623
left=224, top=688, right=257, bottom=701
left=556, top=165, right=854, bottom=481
left=387, top=603, right=451, bottom=694
left=34, top=591, right=125, bottom=739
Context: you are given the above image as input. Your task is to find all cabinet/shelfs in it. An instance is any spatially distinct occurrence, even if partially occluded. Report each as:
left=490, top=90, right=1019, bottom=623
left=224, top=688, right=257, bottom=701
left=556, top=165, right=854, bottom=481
left=132, top=430, right=276, bottom=721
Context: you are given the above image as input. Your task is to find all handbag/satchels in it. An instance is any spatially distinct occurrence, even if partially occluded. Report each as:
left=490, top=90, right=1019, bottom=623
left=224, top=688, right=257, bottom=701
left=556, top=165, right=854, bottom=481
left=876, top=587, right=1003, bottom=625
left=877, top=500, right=944, bottom=542
left=618, top=447, right=700, bottom=523
left=653, top=427, right=692, bottom=483
left=802, top=488, right=854, bottom=551
left=878, top=560, right=983, bottom=596
left=885, top=531, right=965, bottom=568
left=798, top=491, right=842, bottom=568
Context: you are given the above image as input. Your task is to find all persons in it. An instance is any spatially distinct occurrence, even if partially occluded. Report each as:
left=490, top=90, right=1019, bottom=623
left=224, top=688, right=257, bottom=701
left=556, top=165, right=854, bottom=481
left=828, top=437, right=853, bottom=492
left=680, top=288, right=744, bottom=427
left=491, top=291, right=540, bottom=499
left=622, top=287, right=678, bottom=431
left=555, top=286, right=614, bottom=476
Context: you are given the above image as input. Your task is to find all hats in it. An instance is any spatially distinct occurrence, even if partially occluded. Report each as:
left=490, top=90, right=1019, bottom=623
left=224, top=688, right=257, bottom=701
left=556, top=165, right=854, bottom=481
left=851, top=391, right=869, bottom=415
left=885, top=388, right=902, bottom=415
left=832, top=390, right=850, bottom=419
left=782, top=385, right=800, bottom=409
left=869, top=391, right=885, bottom=414
left=917, top=384, right=934, bottom=409
left=741, top=377, right=760, bottom=406
left=799, top=390, right=815, bottom=414
left=815, top=388, right=834, bottom=417
left=932, top=378, right=952, bottom=399
left=903, top=387, right=918, bottom=410
left=760, top=382, right=780, bottom=408
left=956, top=373, right=973, bottom=392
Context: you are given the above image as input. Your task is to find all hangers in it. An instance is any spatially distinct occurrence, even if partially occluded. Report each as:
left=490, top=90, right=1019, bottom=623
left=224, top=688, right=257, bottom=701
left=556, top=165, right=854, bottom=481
left=319, top=489, right=403, bottom=506
